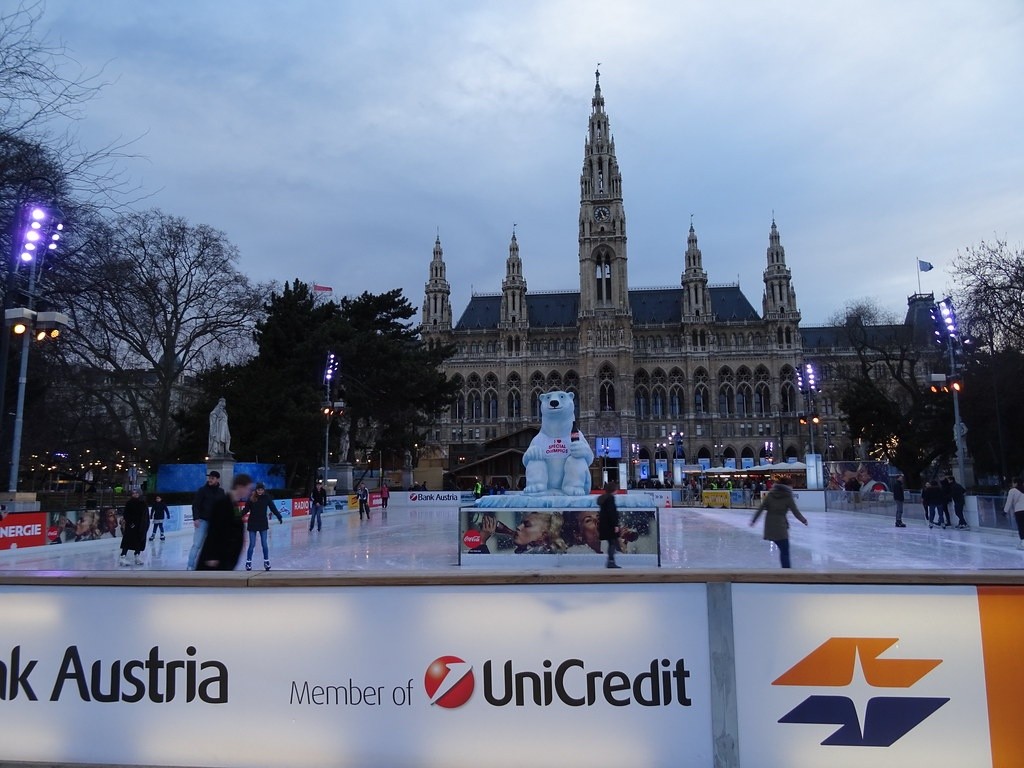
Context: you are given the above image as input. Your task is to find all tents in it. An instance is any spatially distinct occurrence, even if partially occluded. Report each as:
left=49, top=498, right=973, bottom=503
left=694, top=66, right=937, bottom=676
left=702, top=461, right=807, bottom=488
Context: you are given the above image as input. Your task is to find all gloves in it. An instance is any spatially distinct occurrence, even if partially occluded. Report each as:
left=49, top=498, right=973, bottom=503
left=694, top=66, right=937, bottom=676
left=279, top=518, right=282, bottom=524
left=1001, top=511, right=1006, bottom=516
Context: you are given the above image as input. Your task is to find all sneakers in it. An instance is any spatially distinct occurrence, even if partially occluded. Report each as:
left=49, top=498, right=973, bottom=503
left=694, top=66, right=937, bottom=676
left=118, top=554, right=144, bottom=567
left=895, top=522, right=906, bottom=529
left=264, top=561, right=271, bottom=571
left=607, top=562, right=621, bottom=569
left=955, top=524, right=970, bottom=531
left=246, top=562, right=252, bottom=571
left=925, top=518, right=952, bottom=530
left=148, top=533, right=166, bottom=542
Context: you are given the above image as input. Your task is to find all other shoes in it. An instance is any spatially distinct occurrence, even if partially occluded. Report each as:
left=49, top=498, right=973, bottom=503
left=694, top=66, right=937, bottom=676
left=382, top=507, right=388, bottom=510
left=308, top=528, right=321, bottom=533
left=187, top=566, right=192, bottom=570
left=1016, top=540, right=1024, bottom=550
left=359, top=515, right=370, bottom=520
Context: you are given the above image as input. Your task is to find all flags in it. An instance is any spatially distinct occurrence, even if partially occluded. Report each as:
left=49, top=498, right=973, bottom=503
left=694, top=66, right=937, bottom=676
left=314, top=285, right=332, bottom=297
left=919, top=260, right=933, bottom=272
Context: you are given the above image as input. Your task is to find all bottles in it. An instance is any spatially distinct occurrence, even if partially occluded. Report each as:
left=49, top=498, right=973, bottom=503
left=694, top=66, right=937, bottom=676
left=473, top=513, right=517, bottom=537
left=619, top=525, right=638, bottom=542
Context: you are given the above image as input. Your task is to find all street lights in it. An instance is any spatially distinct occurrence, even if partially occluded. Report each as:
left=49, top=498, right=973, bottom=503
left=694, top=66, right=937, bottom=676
left=3, top=204, right=77, bottom=494
left=929, top=297, right=975, bottom=493
left=794, top=362, right=826, bottom=491
left=319, top=350, right=347, bottom=495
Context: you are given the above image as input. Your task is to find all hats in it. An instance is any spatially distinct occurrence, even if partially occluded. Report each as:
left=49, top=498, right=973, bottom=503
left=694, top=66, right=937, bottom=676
left=206, top=471, right=220, bottom=478
left=256, top=484, right=264, bottom=489
left=414, top=481, right=418, bottom=484
left=605, top=482, right=618, bottom=493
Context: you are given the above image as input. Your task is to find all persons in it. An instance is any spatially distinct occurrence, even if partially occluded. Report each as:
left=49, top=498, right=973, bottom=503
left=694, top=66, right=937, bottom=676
left=749, top=476, right=808, bottom=568
left=309, top=482, right=327, bottom=533
left=608, top=473, right=774, bottom=502
left=355, top=483, right=370, bottom=520
left=380, top=483, right=390, bottom=510
left=187, top=471, right=283, bottom=571
left=208, top=398, right=235, bottom=455
left=1002, top=476, right=1024, bottom=550
left=50, top=507, right=126, bottom=545
left=954, top=416, right=969, bottom=459
left=893, top=475, right=906, bottom=527
left=597, top=482, right=623, bottom=569
left=118, top=486, right=150, bottom=567
left=922, top=471, right=970, bottom=530
left=474, top=479, right=506, bottom=500
left=468, top=511, right=630, bottom=555
left=825, top=462, right=893, bottom=503
left=408, top=481, right=426, bottom=491
left=148, top=495, right=170, bottom=542
